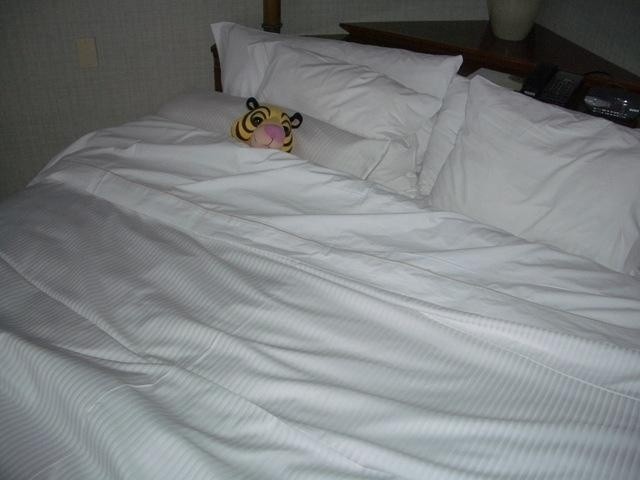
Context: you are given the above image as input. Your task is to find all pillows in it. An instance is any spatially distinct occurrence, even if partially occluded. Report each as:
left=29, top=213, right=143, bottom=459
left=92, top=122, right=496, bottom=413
left=423, top=73, right=639, bottom=271
left=210, top=19, right=468, bottom=200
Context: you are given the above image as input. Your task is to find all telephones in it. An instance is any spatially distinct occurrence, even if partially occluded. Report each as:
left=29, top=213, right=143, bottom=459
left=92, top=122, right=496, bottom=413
left=520, top=62, right=590, bottom=111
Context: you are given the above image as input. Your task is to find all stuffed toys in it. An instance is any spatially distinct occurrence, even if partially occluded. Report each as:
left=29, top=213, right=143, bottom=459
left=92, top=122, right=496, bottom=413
left=231, top=96, right=303, bottom=152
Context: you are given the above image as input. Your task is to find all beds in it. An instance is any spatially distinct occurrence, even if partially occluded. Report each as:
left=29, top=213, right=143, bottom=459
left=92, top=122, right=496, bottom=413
left=0, top=19, right=639, bottom=479
